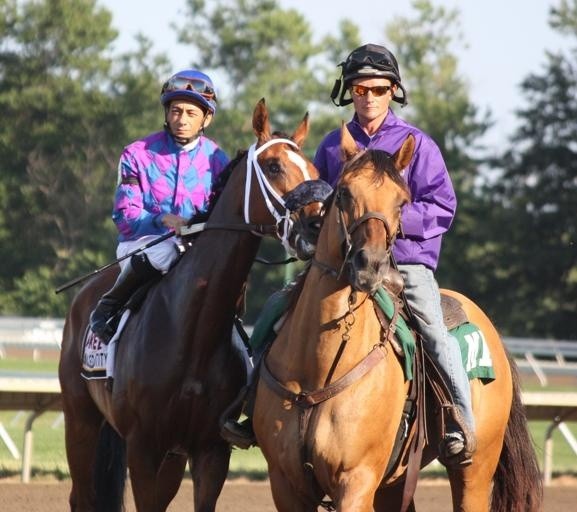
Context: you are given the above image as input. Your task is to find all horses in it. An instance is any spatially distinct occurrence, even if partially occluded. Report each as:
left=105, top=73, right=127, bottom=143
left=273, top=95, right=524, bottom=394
left=254, top=118, right=546, bottom=512
left=56, top=98, right=328, bottom=512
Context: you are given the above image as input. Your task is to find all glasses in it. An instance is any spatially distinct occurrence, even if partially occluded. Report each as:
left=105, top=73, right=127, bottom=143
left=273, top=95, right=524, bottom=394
left=162, top=76, right=217, bottom=101
left=345, top=83, right=394, bottom=97
left=344, top=48, right=400, bottom=73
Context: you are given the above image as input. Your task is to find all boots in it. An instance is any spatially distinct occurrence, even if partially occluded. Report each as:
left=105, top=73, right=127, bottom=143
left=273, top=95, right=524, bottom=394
left=89, top=253, right=161, bottom=346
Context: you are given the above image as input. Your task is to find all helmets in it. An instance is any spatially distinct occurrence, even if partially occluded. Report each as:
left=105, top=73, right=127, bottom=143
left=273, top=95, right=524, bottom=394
left=160, top=70, right=218, bottom=116
left=341, top=44, right=401, bottom=84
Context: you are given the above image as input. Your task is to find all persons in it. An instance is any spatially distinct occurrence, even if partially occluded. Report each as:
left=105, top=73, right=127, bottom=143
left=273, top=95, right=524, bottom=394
left=88, top=70, right=231, bottom=347
left=219, top=41, right=476, bottom=469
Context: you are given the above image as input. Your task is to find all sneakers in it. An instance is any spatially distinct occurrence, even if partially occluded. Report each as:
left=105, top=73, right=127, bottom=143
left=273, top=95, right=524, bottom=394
left=222, top=416, right=258, bottom=446
left=443, top=438, right=473, bottom=468
